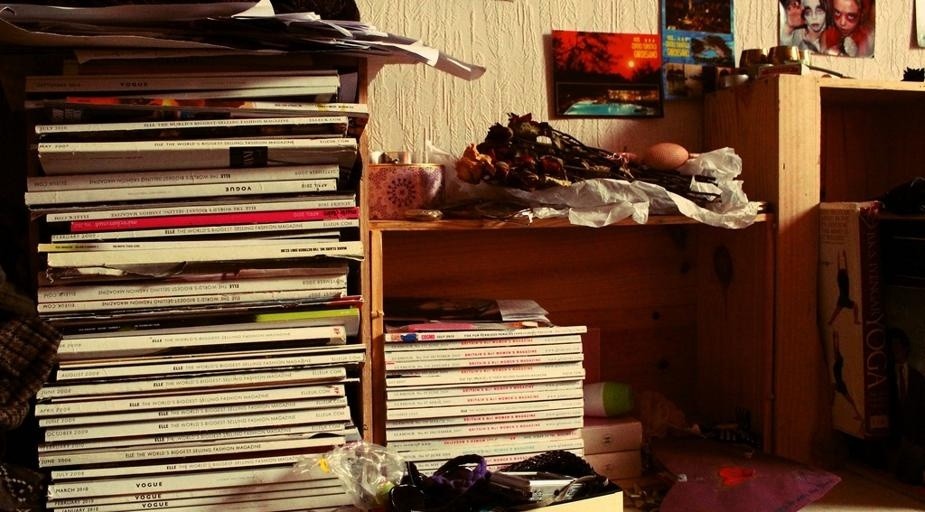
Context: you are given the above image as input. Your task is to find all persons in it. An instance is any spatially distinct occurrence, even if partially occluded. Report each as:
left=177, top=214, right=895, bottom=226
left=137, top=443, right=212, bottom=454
left=779, top=0, right=829, bottom=54
left=827, top=247, right=861, bottom=326
left=820, top=1, right=874, bottom=57
left=828, top=329, right=863, bottom=421
left=666, top=1, right=734, bottom=95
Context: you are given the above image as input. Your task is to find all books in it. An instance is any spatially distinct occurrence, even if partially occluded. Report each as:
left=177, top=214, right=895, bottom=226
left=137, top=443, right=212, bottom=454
left=0, top=0, right=369, bottom=511
left=382, top=297, right=644, bottom=512
